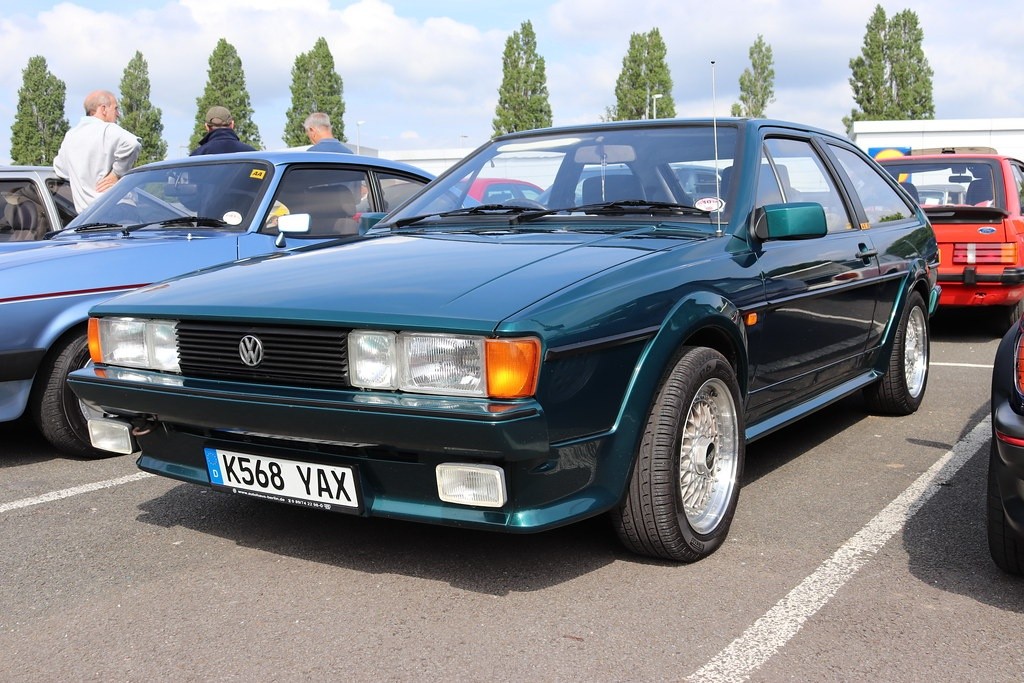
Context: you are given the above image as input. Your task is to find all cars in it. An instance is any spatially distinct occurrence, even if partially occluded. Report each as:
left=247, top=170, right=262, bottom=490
left=0, top=166, right=192, bottom=247
left=990, top=304, right=1024, bottom=577
left=64, top=114, right=939, bottom=563
left=535, top=165, right=728, bottom=214
left=0, top=150, right=482, bottom=460
left=353, top=178, right=544, bottom=223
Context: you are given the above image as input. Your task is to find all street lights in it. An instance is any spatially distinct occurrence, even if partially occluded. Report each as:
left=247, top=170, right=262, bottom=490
left=652, top=93, right=663, bottom=119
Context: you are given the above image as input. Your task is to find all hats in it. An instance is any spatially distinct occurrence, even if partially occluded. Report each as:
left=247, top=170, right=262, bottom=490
left=205, top=106, right=231, bottom=126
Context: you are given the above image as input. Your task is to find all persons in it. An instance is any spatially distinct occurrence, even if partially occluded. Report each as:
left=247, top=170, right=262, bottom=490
left=304, top=112, right=362, bottom=205
left=53, top=89, right=142, bottom=228
left=180, top=105, right=257, bottom=216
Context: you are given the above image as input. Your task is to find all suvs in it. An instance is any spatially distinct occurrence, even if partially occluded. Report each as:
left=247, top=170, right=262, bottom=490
left=866, top=156, right=1024, bottom=333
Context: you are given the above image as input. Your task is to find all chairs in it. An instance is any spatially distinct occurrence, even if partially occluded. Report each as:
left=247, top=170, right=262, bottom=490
left=898, top=182, right=919, bottom=204
left=299, top=181, right=359, bottom=236
left=581, top=171, right=645, bottom=214
left=220, top=179, right=272, bottom=232
left=719, top=163, right=792, bottom=219
left=12, top=199, right=38, bottom=240
left=963, top=178, right=992, bottom=205
